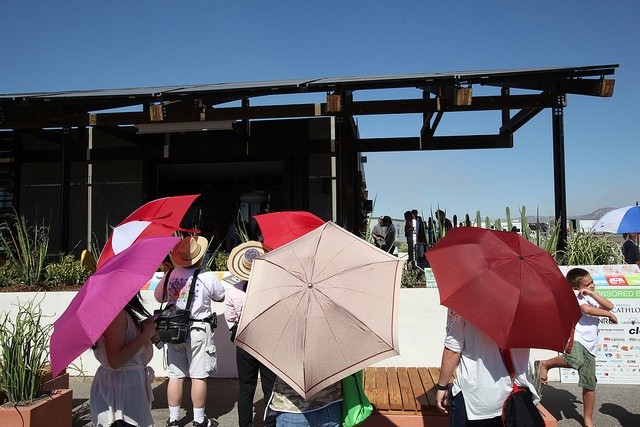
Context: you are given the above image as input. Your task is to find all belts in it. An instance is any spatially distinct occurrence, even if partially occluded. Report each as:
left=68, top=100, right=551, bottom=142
left=189, top=318, right=209, bottom=322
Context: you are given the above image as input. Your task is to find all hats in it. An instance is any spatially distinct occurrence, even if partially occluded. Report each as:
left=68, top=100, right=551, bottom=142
left=227, top=241, right=273, bottom=281
left=172, top=236, right=208, bottom=267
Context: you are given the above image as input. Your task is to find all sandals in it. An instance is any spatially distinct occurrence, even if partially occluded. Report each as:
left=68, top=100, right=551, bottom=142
left=166, top=417, right=180, bottom=427
left=193, top=416, right=219, bottom=427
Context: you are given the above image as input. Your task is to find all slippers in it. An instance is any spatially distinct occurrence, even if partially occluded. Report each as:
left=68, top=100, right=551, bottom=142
left=535, top=360, right=547, bottom=381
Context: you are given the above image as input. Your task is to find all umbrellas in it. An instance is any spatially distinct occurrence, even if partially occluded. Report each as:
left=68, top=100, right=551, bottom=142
left=423, top=227, right=582, bottom=354
left=95, top=194, right=200, bottom=271
left=252, top=211, right=327, bottom=250
left=50, top=236, right=182, bottom=379
left=591, top=201, right=640, bottom=233
left=234, top=221, right=406, bottom=402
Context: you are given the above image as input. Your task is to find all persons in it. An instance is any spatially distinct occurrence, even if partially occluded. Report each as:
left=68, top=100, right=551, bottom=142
left=621, top=233, right=640, bottom=268
left=403, top=211, right=414, bottom=262
left=534, top=268, right=618, bottom=427
left=371, top=216, right=388, bottom=250
left=382, top=215, right=396, bottom=254
left=154, top=236, right=226, bottom=427
left=263, top=376, right=345, bottom=427
left=89, top=291, right=160, bottom=427
left=435, top=210, right=452, bottom=233
left=411, top=209, right=427, bottom=262
left=225, top=241, right=277, bottom=427
left=435, top=307, right=541, bottom=426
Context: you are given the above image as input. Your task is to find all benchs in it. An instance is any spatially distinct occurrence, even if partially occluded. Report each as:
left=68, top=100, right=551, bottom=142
left=363, top=367, right=558, bottom=427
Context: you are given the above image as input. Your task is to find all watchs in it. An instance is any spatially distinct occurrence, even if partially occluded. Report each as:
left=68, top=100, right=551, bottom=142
left=435, top=385, right=450, bottom=390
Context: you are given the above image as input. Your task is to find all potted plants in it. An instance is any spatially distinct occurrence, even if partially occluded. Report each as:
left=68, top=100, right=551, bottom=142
left=0, top=287, right=73, bottom=427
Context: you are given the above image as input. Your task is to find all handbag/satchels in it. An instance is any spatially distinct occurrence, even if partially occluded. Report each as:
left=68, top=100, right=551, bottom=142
left=209, top=312, right=217, bottom=330
left=154, top=305, right=191, bottom=344
left=502, top=387, right=545, bottom=427
left=342, top=369, right=373, bottom=427
